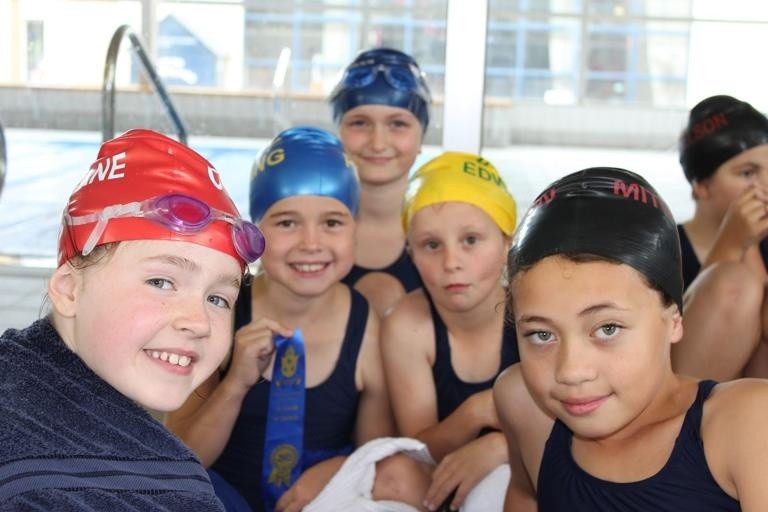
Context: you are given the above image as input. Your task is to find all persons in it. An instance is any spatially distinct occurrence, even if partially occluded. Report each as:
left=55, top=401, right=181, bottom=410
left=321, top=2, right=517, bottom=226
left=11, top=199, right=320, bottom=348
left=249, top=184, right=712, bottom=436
left=368, top=151, right=519, bottom=510
left=491, top=167, right=767, bottom=512
left=326, top=47, right=429, bottom=323
left=163, top=126, right=394, bottom=512
left=0, top=129, right=244, bottom=512
left=667, top=95, right=768, bottom=383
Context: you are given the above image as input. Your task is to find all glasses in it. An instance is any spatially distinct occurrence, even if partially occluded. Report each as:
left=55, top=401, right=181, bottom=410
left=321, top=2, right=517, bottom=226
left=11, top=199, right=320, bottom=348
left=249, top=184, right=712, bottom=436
left=344, top=63, right=422, bottom=92
left=63, top=194, right=265, bottom=264
